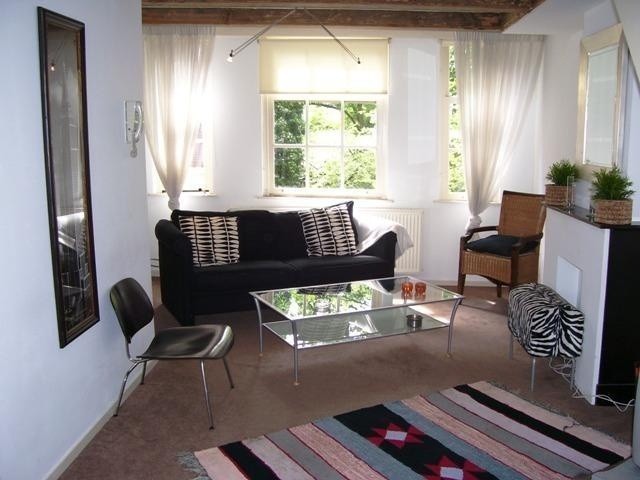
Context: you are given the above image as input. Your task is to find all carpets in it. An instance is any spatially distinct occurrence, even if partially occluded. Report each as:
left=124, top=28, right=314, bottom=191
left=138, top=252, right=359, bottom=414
left=177, top=380, right=632, bottom=480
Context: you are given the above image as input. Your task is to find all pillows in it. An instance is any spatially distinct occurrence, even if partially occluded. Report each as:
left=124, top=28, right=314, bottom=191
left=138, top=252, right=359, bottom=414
left=178, top=216, right=239, bottom=268
left=465, top=235, right=538, bottom=257
left=297, top=204, right=357, bottom=258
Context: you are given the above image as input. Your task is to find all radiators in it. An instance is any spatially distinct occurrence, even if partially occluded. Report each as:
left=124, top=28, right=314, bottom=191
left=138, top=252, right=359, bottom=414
left=361, top=208, right=424, bottom=273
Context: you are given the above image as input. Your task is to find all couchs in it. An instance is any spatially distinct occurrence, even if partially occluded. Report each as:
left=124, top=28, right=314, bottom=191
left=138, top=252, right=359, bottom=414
left=155, top=210, right=414, bottom=326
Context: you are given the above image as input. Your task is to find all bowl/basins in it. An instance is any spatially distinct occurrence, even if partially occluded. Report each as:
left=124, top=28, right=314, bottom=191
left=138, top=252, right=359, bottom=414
left=405, top=313, right=424, bottom=328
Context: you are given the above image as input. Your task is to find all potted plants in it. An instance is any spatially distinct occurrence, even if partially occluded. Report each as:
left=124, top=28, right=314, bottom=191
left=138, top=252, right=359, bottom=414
left=545, top=160, right=582, bottom=207
left=590, top=162, right=636, bottom=226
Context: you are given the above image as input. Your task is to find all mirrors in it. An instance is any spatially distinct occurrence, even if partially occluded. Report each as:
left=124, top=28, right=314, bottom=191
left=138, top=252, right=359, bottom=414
left=37, top=6, right=100, bottom=348
left=577, top=21, right=628, bottom=182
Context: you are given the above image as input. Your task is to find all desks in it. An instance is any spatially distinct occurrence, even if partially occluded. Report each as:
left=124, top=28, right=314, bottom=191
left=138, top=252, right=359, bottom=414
left=249, top=275, right=466, bottom=386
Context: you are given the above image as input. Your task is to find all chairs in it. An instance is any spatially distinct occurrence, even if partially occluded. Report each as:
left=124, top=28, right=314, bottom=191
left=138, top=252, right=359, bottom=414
left=457, top=191, right=546, bottom=305
left=110, top=278, right=234, bottom=431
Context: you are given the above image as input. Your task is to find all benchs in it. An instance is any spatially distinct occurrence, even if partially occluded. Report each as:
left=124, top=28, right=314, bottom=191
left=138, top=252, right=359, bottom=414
left=507, top=283, right=585, bottom=391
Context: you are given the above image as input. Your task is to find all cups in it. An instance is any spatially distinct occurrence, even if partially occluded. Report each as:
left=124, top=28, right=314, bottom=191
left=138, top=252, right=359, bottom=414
left=402, top=282, right=413, bottom=293
left=401, top=292, right=413, bottom=300
left=414, top=293, right=426, bottom=301
left=415, top=282, right=426, bottom=293
